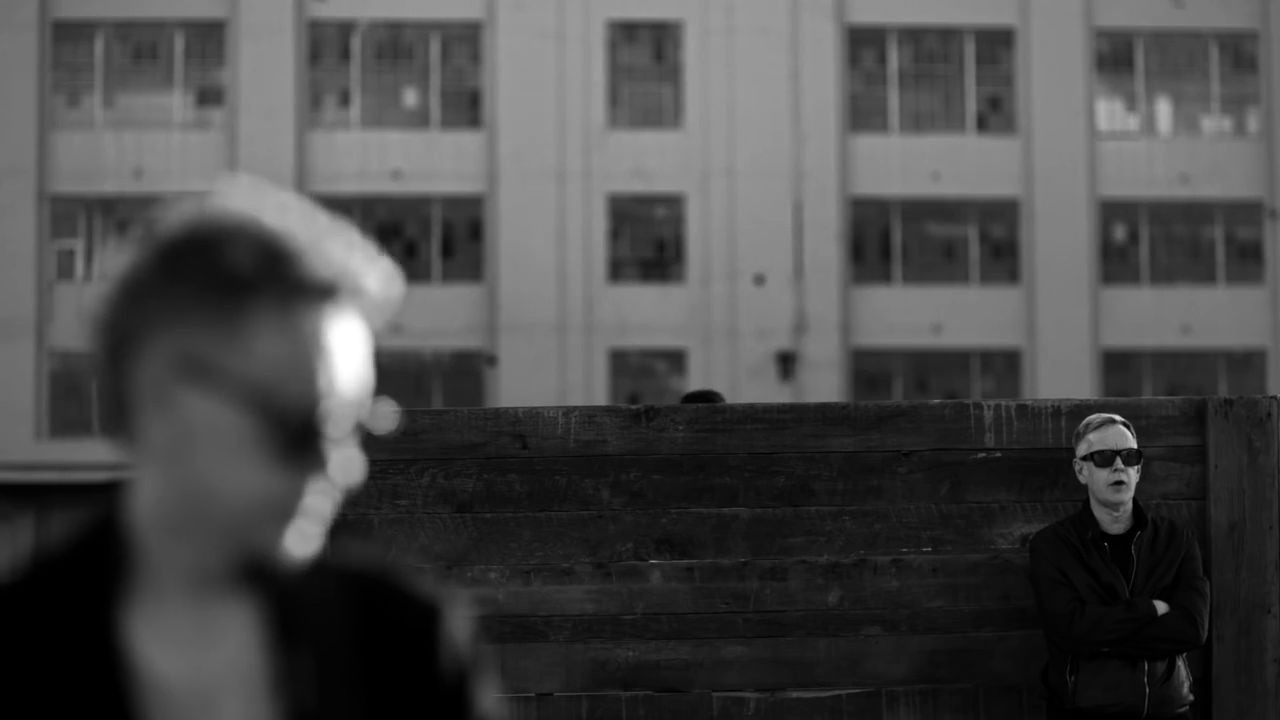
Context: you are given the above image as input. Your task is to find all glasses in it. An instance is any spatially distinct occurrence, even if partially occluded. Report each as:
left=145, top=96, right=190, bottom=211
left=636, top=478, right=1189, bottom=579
left=184, top=357, right=369, bottom=476
left=1079, top=448, right=1144, bottom=468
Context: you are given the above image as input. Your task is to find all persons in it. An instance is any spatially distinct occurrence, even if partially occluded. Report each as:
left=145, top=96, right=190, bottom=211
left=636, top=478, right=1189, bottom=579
left=1029, top=412, right=1209, bottom=719
left=2, top=171, right=502, bottom=718
